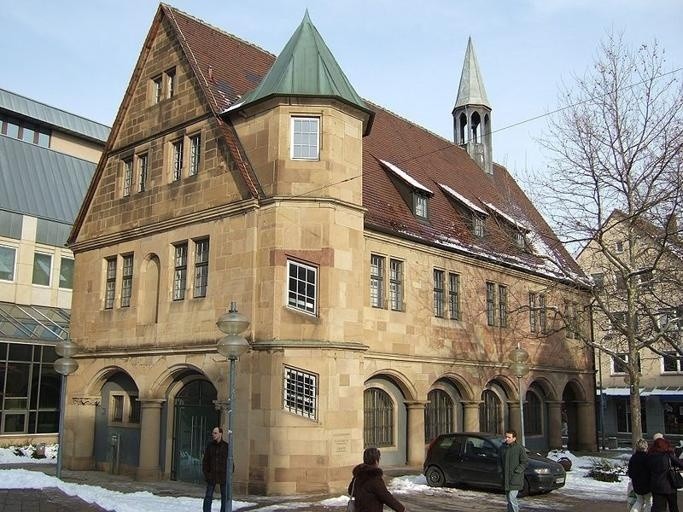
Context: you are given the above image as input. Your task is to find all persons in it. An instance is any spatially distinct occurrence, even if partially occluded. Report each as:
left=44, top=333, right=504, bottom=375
left=346, top=447, right=407, bottom=511
left=201, top=425, right=235, bottom=512
left=496, top=428, right=528, bottom=511
left=643, top=437, right=683, bottom=511
left=626, top=437, right=651, bottom=511
left=560, top=408, right=568, bottom=435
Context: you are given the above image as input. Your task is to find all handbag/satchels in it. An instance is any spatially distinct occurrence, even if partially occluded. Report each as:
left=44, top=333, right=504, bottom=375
left=348, top=478, right=356, bottom=512
left=668, top=452, right=683, bottom=489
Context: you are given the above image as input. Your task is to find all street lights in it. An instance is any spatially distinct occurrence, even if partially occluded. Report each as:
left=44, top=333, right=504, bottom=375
left=216, top=301, right=250, bottom=512
left=598, top=335, right=612, bottom=450
left=507, top=342, right=528, bottom=448
left=53, top=332, right=78, bottom=480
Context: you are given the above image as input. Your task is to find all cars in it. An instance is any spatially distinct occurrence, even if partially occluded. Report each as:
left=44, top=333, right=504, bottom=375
left=423, top=432, right=565, bottom=498
